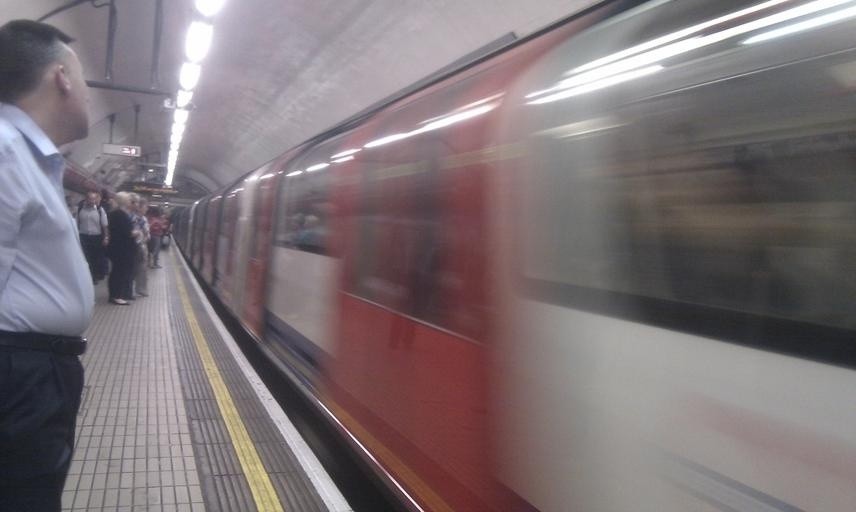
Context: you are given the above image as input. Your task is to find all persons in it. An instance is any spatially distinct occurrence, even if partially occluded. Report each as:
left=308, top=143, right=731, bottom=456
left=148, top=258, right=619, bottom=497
left=0, top=19, right=94, bottom=512
left=66, top=193, right=175, bottom=304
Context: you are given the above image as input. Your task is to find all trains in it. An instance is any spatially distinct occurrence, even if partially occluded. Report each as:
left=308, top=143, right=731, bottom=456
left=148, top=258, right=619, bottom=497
left=63, top=153, right=118, bottom=222
left=172, top=1, right=856, bottom=511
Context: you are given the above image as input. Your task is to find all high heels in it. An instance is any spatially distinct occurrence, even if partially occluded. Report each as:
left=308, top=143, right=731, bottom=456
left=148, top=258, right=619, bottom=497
left=110, top=293, right=136, bottom=305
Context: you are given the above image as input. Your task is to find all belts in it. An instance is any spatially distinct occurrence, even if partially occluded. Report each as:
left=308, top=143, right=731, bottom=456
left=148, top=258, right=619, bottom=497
left=0, top=330, right=87, bottom=356
